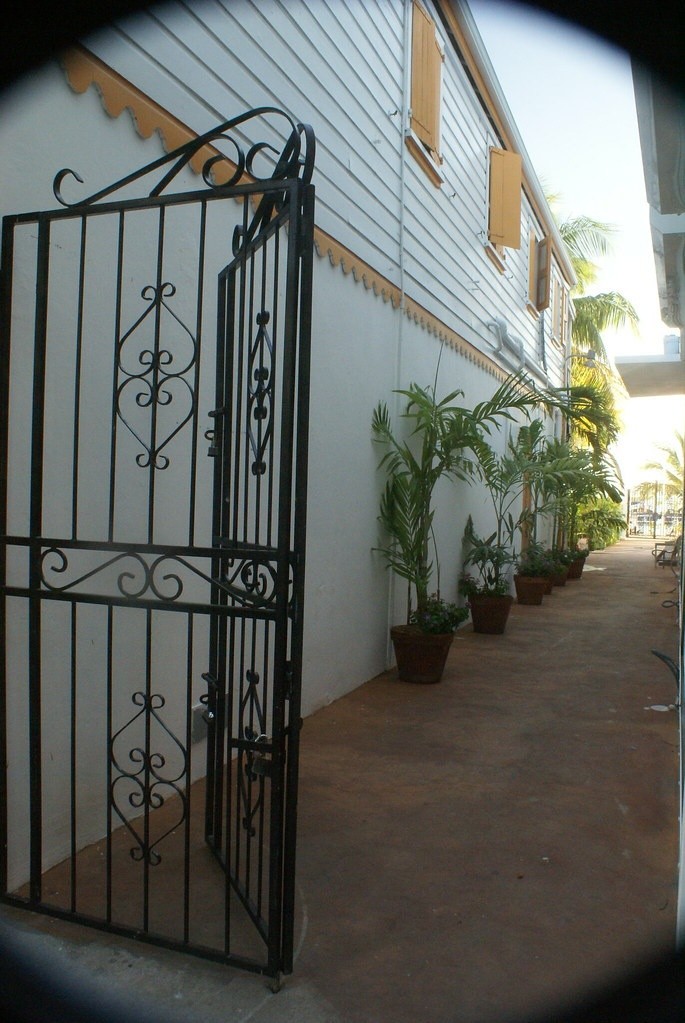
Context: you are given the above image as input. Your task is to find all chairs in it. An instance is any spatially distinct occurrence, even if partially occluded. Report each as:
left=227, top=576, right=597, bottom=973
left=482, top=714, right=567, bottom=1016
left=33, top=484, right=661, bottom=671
left=651, top=535, right=681, bottom=578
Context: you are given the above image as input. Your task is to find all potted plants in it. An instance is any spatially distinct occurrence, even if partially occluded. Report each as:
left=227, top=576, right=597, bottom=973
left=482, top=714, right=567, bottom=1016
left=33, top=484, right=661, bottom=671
left=454, top=430, right=625, bottom=634
left=369, top=341, right=614, bottom=682
left=511, top=434, right=629, bottom=606
